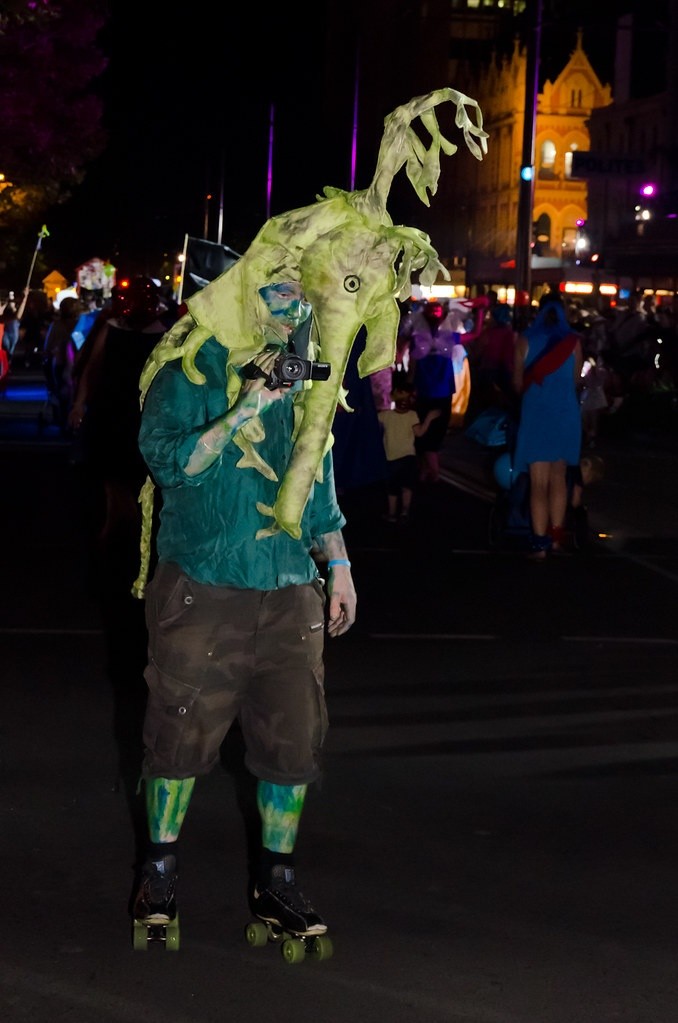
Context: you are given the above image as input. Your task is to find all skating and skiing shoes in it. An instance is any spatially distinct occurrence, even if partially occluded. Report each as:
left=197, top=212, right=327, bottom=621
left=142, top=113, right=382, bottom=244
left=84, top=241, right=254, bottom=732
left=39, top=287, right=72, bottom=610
left=244, top=849, right=333, bottom=966
left=133, top=840, right=183, bottom=951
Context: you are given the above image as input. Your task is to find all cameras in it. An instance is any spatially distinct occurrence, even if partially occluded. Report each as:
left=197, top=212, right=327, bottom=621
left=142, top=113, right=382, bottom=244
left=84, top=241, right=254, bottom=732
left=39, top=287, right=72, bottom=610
left=262, top=344, right=331, bottom=390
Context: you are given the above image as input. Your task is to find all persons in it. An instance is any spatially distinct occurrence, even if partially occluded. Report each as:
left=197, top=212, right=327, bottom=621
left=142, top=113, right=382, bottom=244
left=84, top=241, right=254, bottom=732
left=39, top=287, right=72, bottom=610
left=334, top=288, right=677, bottom=560
left=127, top=274, right=356, bottom=961
left=4, top=277, right=178, bottom=550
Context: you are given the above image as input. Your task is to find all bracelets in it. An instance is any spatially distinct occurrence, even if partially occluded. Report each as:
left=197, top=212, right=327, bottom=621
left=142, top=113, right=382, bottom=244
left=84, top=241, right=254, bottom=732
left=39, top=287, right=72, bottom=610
left=328, top=560, right=351, bottom=569
